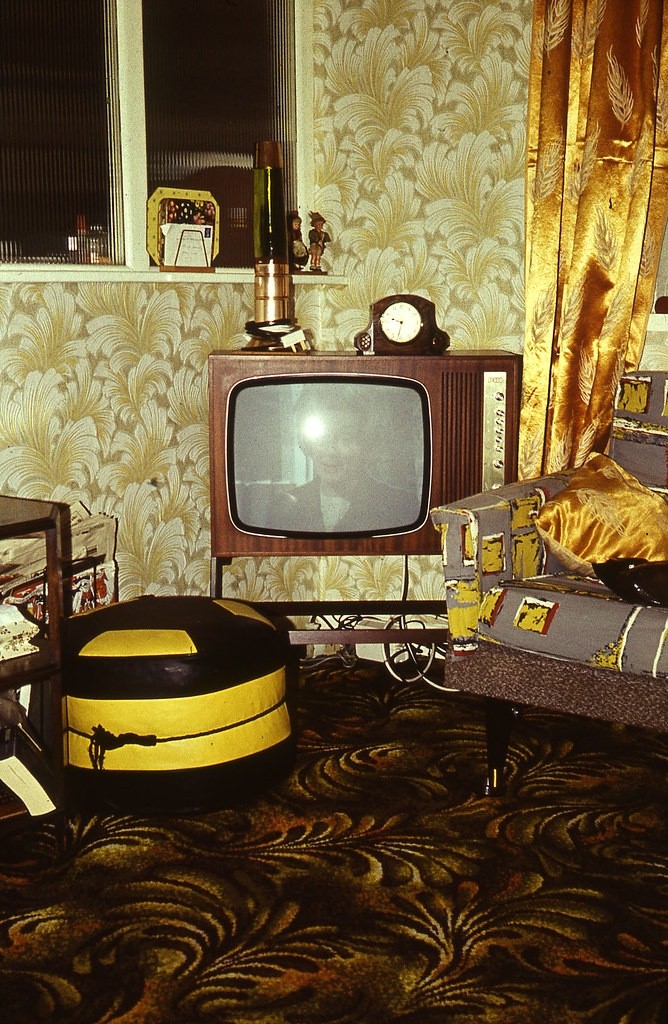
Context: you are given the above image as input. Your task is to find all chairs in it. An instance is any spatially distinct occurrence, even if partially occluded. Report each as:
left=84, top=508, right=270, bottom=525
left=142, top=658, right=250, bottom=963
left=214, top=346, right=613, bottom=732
left=429, top=369, right=668, bottom=799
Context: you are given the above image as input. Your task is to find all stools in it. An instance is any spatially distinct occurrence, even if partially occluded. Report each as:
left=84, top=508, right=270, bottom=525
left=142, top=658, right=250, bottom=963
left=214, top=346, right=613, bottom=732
left=55, top=593, right=293, bottom=809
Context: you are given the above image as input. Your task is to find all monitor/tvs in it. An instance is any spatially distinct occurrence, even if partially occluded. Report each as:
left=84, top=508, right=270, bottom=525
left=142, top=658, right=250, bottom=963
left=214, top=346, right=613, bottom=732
left=209, top=347, right=522, bottom=557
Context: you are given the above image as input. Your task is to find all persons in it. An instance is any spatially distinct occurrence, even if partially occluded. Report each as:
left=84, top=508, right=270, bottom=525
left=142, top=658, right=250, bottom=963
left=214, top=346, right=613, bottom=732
left=308, top=210, right=331, bottom=269
left=289, top=211, right=309, bottom=270
left=274, top=382, right=420, bottom=533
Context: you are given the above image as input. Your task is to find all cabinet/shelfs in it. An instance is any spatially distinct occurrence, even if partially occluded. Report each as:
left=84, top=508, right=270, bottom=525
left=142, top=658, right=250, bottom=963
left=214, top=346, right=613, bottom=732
left=0, top=493, right=72, bottom=821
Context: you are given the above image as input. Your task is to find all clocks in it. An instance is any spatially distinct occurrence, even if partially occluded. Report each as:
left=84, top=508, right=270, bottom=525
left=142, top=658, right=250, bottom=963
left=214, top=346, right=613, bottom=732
left=370, top=295, right=452, bottom=355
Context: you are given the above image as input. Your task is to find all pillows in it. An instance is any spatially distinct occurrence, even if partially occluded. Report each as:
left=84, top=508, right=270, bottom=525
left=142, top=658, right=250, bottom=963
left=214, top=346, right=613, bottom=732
left=534, top=449, right=668, bottom=586
